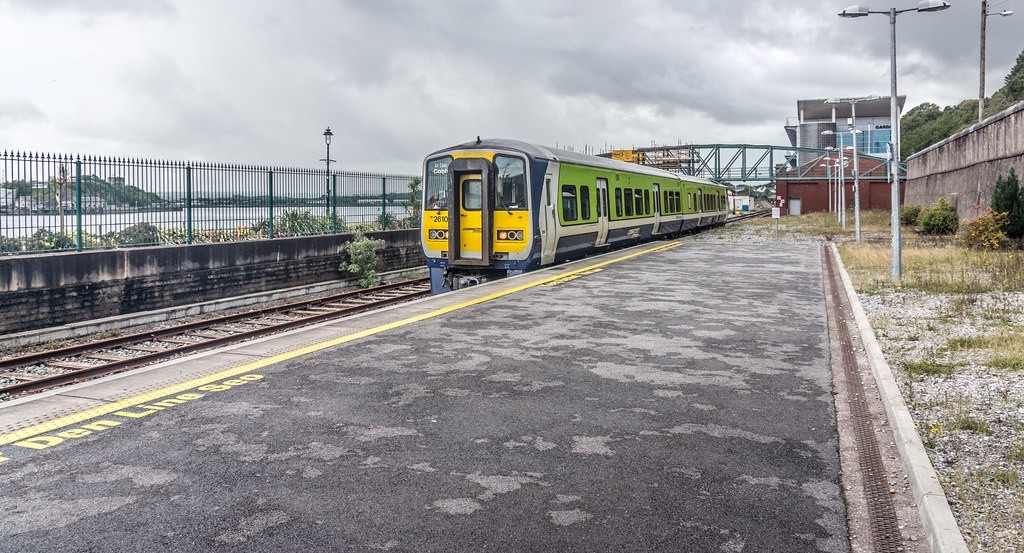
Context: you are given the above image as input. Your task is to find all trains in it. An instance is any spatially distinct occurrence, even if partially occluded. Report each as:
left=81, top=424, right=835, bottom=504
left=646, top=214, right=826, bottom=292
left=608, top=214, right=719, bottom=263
left=417, top=136, right=730, bottom=292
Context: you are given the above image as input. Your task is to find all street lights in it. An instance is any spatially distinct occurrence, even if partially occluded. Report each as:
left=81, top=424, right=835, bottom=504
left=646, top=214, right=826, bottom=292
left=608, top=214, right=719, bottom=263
left=835, top=0, right=952, bottom=281
left=824, top=95, right=883, bottom=243
left=821, top=128, right=864, bottom=230
left=322, top=126, right=334, bottom=222
left=820, top=145, right=854, bottom=224
left=978, top=0, right=1013, bottom=122
